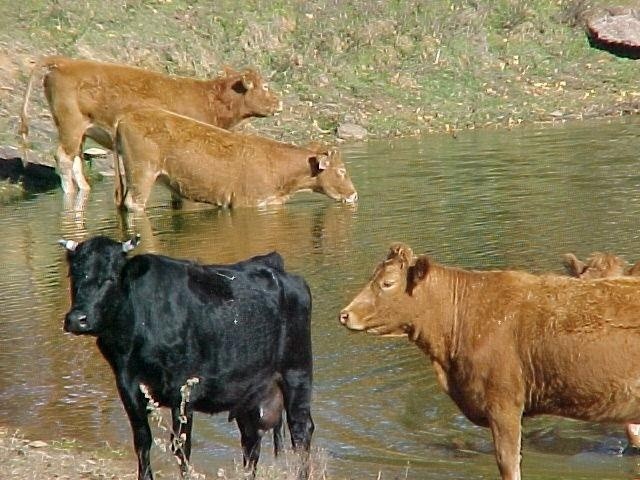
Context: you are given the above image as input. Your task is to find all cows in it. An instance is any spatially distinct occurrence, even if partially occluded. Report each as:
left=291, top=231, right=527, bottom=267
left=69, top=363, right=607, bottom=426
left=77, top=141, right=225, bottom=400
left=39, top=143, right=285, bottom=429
left=558, top=249, right=640, bottom=453
left=110, top=103, right=359, bottom=214
left=17, top=55, right=285, bottom=195
left=49, top=231, right=315, bottom=480
left=338, top=242, right=640, bottom=479
